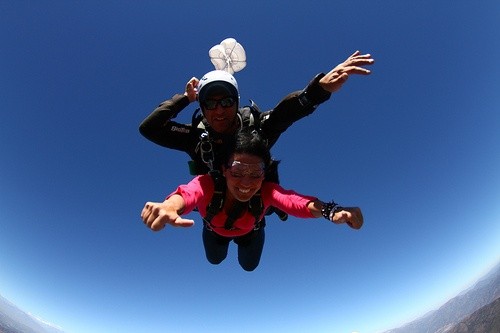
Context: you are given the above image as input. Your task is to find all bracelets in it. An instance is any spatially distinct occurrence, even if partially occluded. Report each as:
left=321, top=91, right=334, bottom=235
left=321, top=200, right=343, bottom=222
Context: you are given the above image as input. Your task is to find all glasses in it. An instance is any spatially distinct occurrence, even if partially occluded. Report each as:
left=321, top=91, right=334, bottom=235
left=201, top=96, right=235, bottom=110
left=225, top=160, right=266, bottom=178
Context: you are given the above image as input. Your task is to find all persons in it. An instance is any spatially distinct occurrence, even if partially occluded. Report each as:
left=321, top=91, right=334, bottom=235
left=139, top=50, right=374, bottom=221
left=141, top=130, right=363, bottom=271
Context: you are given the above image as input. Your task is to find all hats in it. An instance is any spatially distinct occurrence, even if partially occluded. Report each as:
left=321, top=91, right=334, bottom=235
left=198, top=69, right=240, bottom=108
